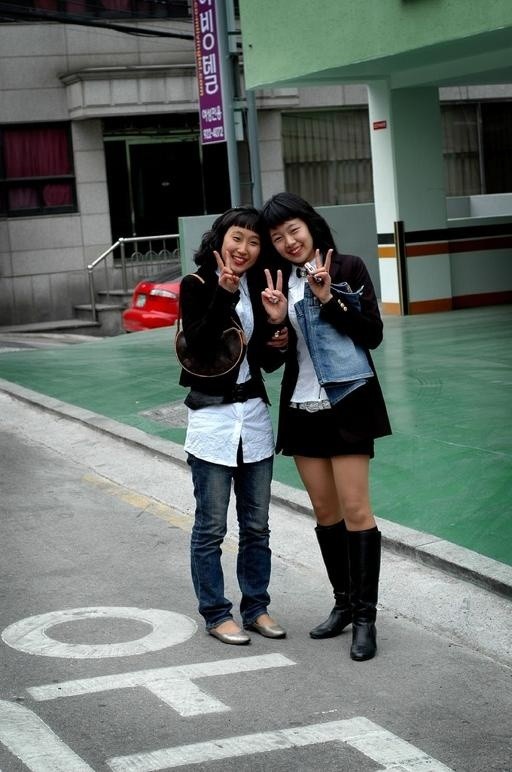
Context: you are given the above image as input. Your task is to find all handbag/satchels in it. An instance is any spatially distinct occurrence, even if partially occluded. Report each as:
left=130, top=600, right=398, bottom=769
left=172, top=321, right=247, bottom=378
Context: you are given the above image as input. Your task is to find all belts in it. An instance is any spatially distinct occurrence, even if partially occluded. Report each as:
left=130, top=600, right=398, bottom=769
left=287, top=400, right=333, bottom=414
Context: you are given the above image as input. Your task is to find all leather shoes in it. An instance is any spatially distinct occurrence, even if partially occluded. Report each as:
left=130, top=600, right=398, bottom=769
left=206, top=618, right=252, bottom=645
left=247, top=615, right=288, bottom=638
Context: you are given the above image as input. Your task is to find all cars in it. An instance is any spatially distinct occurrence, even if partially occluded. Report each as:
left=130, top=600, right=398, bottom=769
left=124, top=268, right=183, bottom=332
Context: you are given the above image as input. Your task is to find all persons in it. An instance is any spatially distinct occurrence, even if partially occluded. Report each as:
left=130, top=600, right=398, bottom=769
left=259, top=191, right=392, bottom=661
left=178, top=205, right=291, bottom=647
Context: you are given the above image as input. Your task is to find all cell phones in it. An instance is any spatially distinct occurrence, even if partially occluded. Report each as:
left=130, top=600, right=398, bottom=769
left=304, top=261, right=322, bottom=283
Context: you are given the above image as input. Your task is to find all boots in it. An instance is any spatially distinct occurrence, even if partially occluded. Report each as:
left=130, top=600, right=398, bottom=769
left=310, top=519, right=353, bottom=640
left=347, top=526, right=384, bottom=660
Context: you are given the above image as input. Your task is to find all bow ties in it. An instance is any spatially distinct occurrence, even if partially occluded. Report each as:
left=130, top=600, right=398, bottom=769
left=295, top=267, right=310, bottom=279
